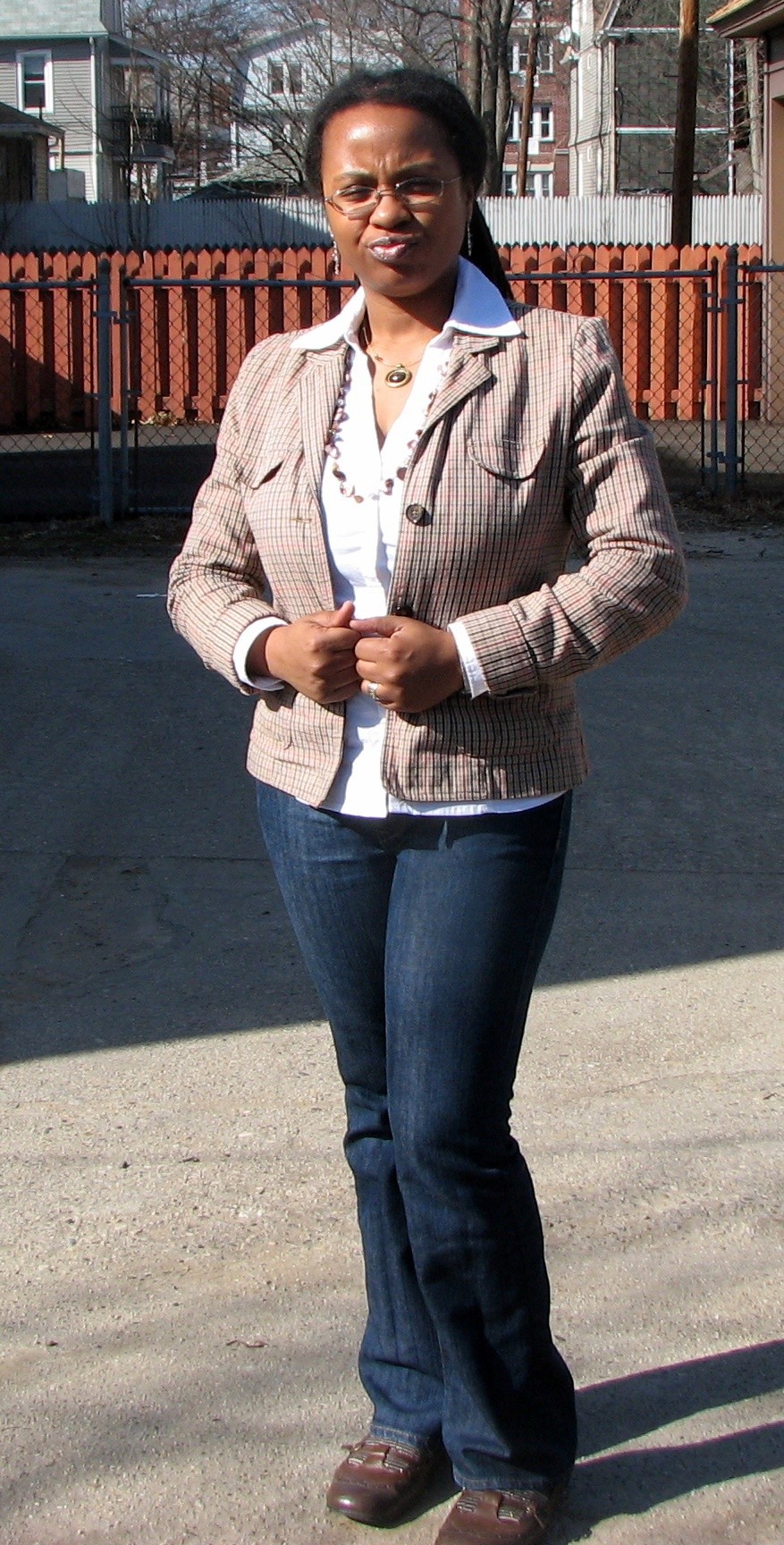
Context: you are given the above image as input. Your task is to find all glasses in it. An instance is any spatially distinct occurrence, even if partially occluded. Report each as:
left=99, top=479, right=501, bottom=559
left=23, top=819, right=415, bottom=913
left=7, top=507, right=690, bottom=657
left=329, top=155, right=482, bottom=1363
left=325, top=177, right=463, bottom=213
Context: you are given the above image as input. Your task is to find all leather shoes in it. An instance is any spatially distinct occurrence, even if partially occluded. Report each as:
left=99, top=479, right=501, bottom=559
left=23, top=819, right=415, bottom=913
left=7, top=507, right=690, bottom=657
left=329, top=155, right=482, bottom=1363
left=328, top=1432, right=443, bottom=1525
left=434, top=1455, right=570, bottom=1545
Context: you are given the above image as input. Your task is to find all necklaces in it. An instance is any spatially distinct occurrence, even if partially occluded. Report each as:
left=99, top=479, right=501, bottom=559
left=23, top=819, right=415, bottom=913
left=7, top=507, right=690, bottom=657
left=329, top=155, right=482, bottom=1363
left=362, top=319, right=422, bottom=388
left=325, top=350, right=449, bottom=504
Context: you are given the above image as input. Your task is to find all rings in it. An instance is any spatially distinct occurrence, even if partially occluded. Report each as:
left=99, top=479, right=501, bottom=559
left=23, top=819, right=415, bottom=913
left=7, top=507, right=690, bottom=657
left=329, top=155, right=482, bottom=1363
left=366, top=682, right=380, bottom=701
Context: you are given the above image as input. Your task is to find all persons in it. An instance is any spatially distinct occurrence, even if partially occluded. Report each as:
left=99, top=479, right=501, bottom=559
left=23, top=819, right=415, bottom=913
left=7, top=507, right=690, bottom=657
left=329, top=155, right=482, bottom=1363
left=169, top=71, right=682, bottom=1545
